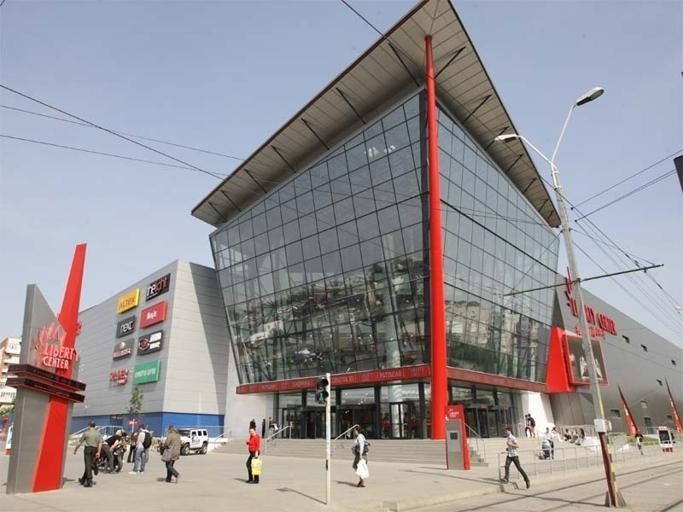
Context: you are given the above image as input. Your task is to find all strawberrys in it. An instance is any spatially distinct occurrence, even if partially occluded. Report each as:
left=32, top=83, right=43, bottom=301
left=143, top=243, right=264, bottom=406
left=94, top=436, right=129, bottom=474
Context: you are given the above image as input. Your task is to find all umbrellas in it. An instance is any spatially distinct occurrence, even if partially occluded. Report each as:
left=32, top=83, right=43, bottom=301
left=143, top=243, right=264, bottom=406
left=79, top=478, right=95, bottom=486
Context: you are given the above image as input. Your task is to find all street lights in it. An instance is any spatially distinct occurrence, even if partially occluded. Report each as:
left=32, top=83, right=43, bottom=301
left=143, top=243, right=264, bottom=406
left=355, top=445, right=368, bottom=456
left=250, top=456, right=262, bottom=475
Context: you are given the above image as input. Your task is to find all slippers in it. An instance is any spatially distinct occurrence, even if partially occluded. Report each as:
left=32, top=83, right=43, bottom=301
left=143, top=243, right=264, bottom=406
left=142, top=431, right=152, bottom=448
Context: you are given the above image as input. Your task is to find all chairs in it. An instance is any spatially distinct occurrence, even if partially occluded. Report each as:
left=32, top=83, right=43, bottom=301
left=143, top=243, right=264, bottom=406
left=357, top=481, right=367, bottom=486
left=247, top=479, right=259, bottom=484
left=129, top=471, right=135, bottom=474
left=526, top=481, right=530, bottom=489
left=175, top=474, right=180, bottom=484
left=499, top=478, right=508, bottom=483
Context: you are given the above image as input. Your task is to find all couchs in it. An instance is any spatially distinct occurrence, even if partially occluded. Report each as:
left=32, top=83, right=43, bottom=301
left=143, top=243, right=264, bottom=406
left=316, top=378, right=329, bottom=403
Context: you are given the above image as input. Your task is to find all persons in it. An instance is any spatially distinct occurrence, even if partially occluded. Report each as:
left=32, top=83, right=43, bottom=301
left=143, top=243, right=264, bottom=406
left=403, top=412, right=411, bottom=438
left=244, top=427, right=261, bottom=484
left=635, top=430, right=644, bottom=455
left=351, top=426, right=370, bottom=487
left=499, top=427, right=530, bottom=489
left=541, top=423, right=585, bottom=461
left=524, top=414, right=537, bottom=439
left=73, top=420, right=182, bottom=487
left=382, top=412, right=393, bottom=439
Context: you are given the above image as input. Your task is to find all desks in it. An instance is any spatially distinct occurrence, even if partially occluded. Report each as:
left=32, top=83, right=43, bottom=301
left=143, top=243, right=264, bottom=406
left=494, top=93, right=628, bottom=508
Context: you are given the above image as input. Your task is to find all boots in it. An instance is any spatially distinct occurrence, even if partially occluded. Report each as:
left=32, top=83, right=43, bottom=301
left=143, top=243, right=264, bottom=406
left=157, top=427, right=209, bottom=456
